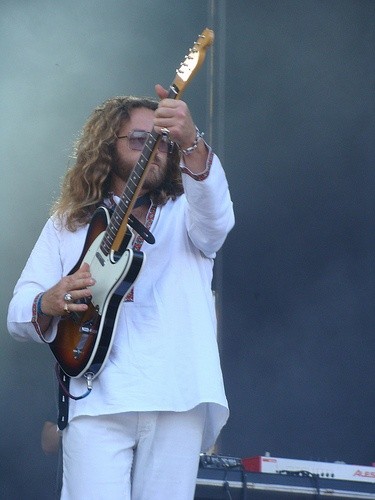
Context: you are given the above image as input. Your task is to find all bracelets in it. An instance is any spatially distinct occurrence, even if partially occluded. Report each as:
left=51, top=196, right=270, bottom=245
left=177, top=127, right=200, bottom=154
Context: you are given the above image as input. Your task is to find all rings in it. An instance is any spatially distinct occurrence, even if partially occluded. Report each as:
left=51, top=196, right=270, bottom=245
left=64, top=293, right=72, bottom=314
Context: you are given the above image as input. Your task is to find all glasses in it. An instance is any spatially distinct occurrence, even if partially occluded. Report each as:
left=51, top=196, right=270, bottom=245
left=117, top=128, right=171, bottom=153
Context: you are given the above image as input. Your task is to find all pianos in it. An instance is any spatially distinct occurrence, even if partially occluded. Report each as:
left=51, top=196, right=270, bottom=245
left=191, top=477, right=375, bottom=500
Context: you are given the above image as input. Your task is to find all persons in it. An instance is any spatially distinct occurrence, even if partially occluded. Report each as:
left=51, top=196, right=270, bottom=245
left=7, top=85, right=236, bottom=500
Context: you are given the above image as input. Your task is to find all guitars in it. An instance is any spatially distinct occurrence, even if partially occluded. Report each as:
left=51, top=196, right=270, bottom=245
left=47, top=27, right=215, bottom=382
